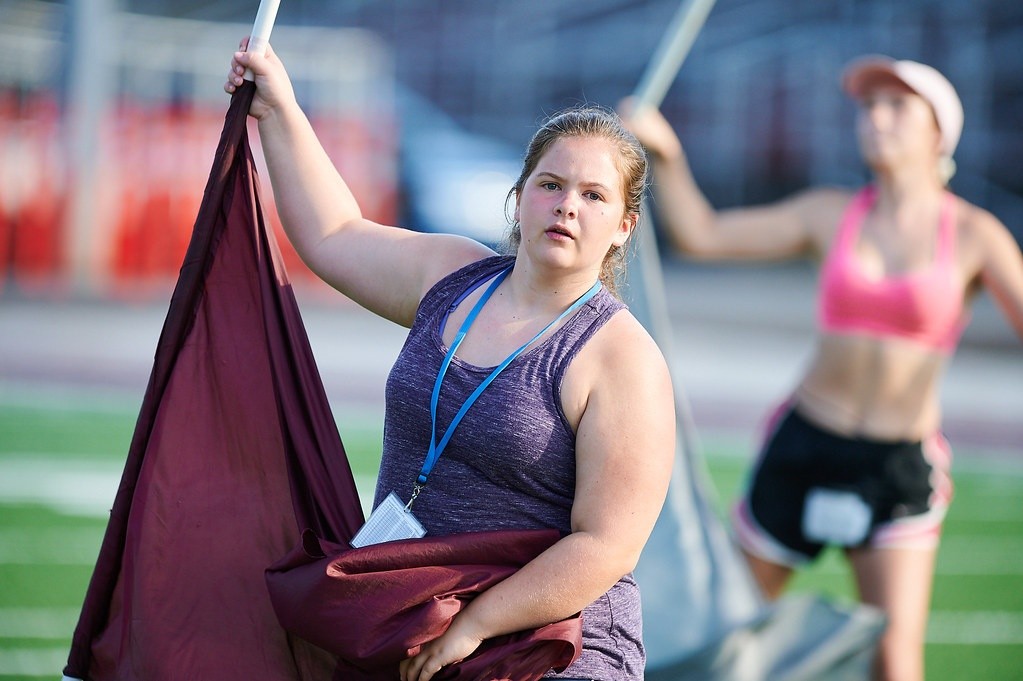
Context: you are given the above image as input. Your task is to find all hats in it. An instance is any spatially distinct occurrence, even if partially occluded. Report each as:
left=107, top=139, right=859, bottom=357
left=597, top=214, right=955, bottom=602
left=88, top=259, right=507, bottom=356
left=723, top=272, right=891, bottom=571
left=844, top=59, right=962, bottom=156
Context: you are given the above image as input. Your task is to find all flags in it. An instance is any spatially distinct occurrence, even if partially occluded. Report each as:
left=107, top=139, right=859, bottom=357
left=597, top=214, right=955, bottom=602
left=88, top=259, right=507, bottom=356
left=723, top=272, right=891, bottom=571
left=61, top=78, right=584, bottom=680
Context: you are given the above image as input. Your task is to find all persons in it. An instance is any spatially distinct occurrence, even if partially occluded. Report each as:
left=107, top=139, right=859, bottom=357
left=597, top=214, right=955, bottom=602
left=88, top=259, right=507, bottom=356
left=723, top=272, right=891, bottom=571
left=226, top=31, right=675, bottom=681
left=614, top=52, right=1023, bottom=681
